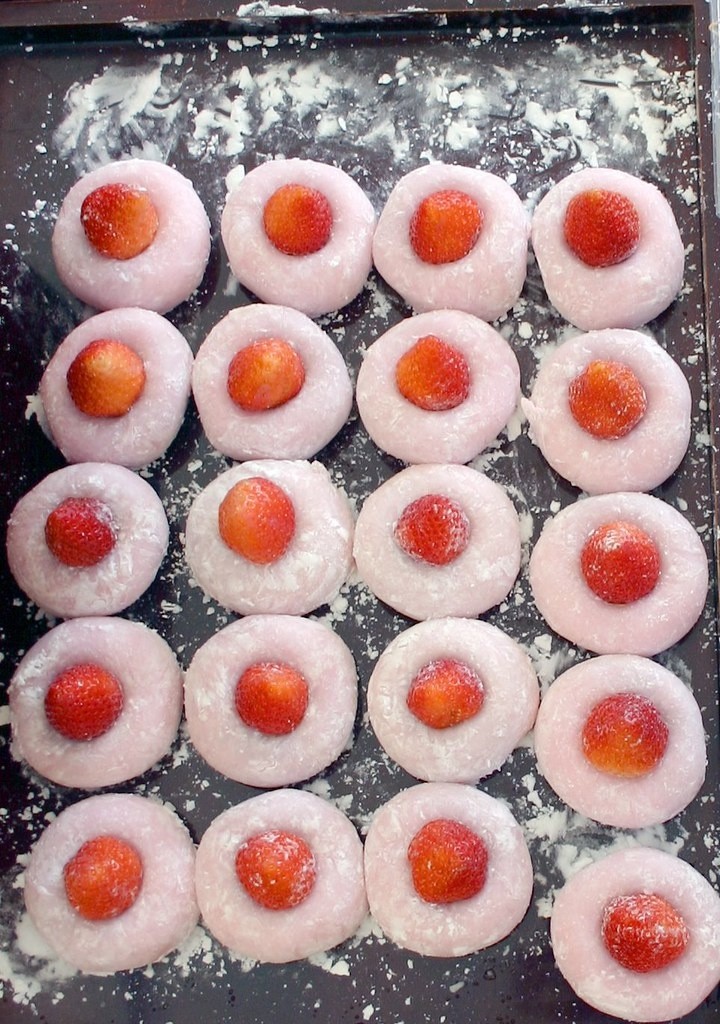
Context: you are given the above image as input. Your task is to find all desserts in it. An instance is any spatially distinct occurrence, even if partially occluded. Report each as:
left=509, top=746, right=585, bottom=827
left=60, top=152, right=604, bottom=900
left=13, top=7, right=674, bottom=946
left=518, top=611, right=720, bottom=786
left=0, top=159, right=720, bottom=1024
